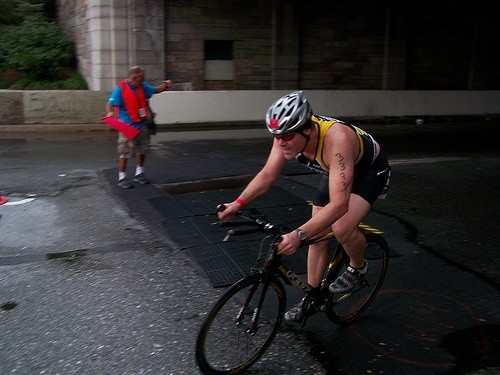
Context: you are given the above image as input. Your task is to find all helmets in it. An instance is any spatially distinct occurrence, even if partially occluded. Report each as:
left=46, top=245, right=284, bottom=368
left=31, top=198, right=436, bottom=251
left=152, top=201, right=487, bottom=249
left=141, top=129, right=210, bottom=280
left=265, top=90, right=312, bottom=134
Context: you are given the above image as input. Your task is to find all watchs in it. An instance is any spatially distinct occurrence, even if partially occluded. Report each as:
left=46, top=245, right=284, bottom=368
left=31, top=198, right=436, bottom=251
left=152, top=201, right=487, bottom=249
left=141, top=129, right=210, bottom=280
left=296, top=228, right=309, bottom=243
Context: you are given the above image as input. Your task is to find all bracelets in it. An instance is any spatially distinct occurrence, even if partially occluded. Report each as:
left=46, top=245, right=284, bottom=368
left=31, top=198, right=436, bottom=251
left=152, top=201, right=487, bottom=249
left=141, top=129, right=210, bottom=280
left=235, top=198, right=246, bottom=209
left=162, top=81, right=168, bottom=87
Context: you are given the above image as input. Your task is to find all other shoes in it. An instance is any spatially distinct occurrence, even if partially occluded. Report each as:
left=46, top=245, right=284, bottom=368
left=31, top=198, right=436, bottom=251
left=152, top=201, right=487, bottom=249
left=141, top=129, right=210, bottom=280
left=133, top=172, right=150, bottom=184
left=117, top=177, right=134, bottom=189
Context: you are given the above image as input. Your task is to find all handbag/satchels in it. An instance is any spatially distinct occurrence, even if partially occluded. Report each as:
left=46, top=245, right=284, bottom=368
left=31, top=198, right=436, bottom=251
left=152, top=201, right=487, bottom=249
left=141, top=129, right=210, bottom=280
left=141, top=84, right=157, bottom=135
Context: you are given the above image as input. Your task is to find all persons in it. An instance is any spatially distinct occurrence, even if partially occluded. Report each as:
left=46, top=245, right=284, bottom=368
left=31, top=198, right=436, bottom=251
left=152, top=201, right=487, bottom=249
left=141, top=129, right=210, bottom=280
left=218, top=91, right=391, bottom=324
left=106, top=66, right=172, bottom=190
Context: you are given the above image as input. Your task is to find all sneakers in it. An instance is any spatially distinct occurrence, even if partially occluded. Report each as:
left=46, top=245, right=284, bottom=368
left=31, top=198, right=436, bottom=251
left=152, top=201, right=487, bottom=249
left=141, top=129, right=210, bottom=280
left=329, top=259, right=368, bottom=293
left=284, top=298, right=314, bottom=321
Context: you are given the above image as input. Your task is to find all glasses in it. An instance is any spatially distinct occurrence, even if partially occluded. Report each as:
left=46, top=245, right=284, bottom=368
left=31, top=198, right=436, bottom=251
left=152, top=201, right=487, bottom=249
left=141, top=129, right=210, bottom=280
left=271, top=131, right=296, bottom=141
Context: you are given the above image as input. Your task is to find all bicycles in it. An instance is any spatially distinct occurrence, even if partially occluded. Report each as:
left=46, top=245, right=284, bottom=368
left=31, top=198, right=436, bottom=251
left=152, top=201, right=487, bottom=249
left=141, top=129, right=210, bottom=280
left=195, top=204, right=391, bottom=375
